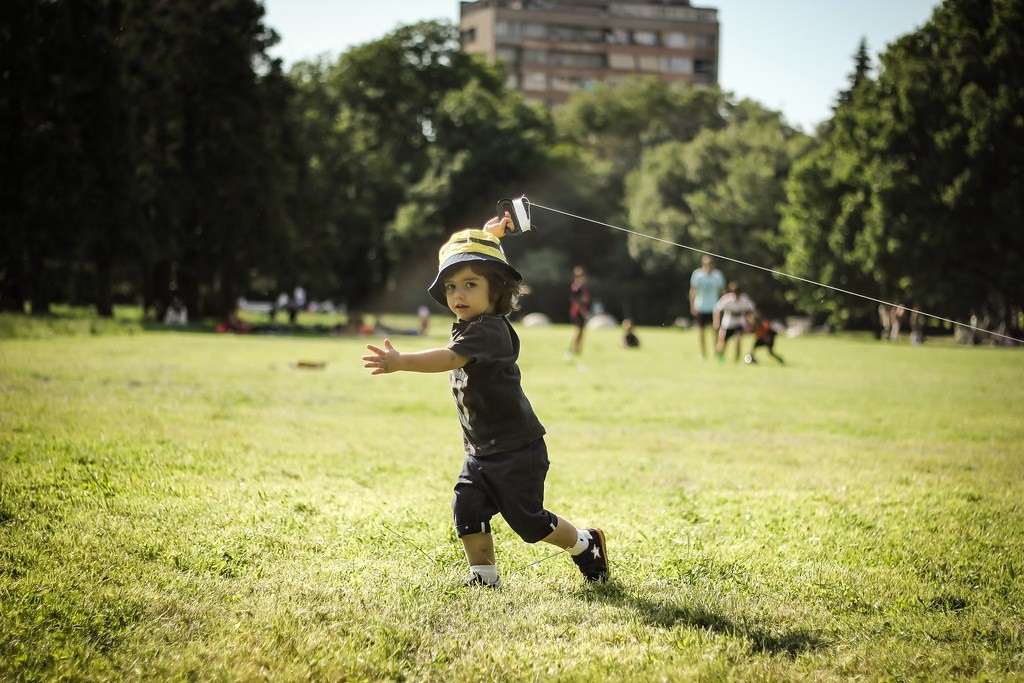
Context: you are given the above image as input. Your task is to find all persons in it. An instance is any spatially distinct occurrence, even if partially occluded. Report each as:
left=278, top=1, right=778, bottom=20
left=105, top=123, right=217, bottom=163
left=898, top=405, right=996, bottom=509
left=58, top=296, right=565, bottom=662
left=747, top=315, right=785, bottom=365
left=714, top=281, right=757, bottom=362
left=689, top=255, right=725, bottom=360
left=162, top=298, right=187, bottom=328
left=417, top=305, right=430, bottom=332
left=269, top=288, right=344, bottom=324
left=968, top=308, right=1024, bottom=345
left=569, top=267, right=593, bottom=355
left=877, top=302, right=925, bottom=344
left=618, top=319, right=639, bottom=348
left=362, top=210, right=609, bottom=585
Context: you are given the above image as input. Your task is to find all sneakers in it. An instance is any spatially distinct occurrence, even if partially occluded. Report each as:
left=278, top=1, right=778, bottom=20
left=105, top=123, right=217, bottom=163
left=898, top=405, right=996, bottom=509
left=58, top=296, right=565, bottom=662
left=573, top=528, right=610, bottom=584
left=463, top=572, right=502, bottom=589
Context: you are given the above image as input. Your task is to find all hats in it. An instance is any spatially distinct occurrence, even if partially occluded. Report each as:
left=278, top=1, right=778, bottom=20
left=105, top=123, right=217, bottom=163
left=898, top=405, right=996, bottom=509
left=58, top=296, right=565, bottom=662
left=428, top=229, right=523, bottom=308
